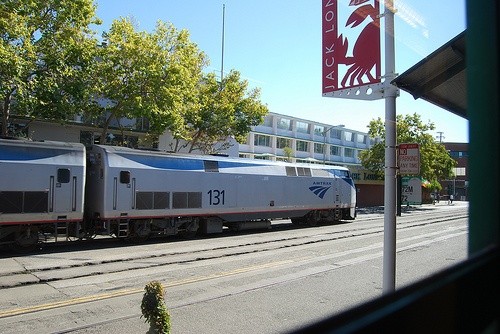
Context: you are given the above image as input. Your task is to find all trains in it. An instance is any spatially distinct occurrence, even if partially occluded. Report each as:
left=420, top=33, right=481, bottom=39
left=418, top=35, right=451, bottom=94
left=0, top=135, right=356, bottom=253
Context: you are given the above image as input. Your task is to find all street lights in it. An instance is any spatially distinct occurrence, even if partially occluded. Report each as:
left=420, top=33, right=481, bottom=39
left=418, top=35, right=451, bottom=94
left=322, top=124, right=345, bottom=164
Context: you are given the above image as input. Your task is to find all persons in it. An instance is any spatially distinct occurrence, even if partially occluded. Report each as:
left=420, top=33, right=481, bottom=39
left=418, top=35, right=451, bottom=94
left=449, top=194, right=454, bottom=204
left=436, top=193, right=441, bottom=203
